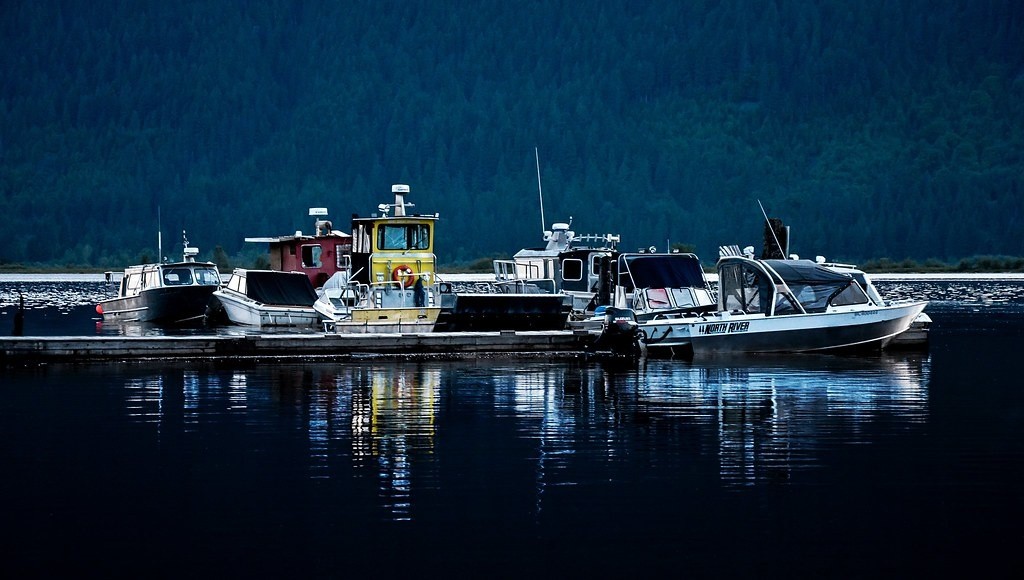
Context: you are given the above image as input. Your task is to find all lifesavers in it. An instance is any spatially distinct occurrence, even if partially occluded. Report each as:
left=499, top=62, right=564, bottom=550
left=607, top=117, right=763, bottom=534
left=393, top=266, right=414, bottom=288
left=317, top=273, right=330, bottom=287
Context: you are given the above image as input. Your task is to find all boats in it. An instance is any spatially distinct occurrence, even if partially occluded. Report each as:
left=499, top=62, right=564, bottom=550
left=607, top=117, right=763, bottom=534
left=566, top=245, right=933, bottom=354
left=96, top=230, right=227, bottom=330
left=244, top=184, right=620, bottom=332
left=213, top=268, right=319, bottom=327
